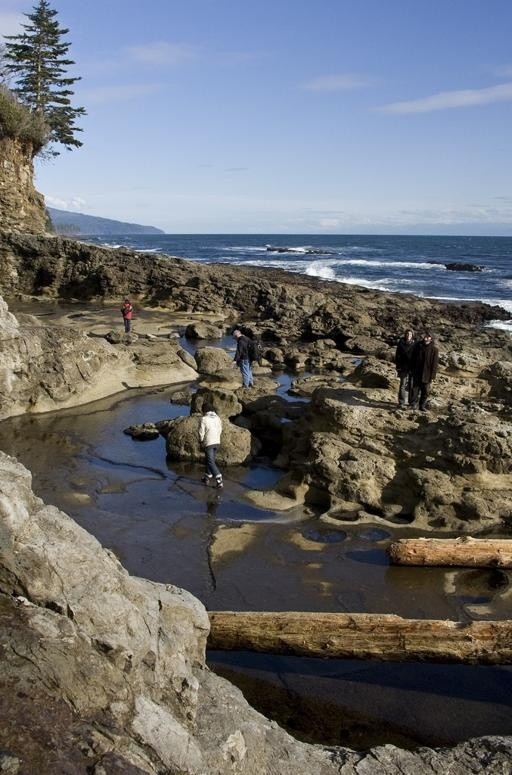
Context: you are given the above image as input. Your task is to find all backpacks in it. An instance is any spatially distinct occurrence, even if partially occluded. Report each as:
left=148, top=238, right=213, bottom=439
left=242, top=338, right=263, bottom=361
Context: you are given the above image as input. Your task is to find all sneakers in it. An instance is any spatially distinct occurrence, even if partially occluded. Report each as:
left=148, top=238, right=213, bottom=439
left=409, top=403, right=426, bottom=411
left=397, top=403, right=403, bottom=407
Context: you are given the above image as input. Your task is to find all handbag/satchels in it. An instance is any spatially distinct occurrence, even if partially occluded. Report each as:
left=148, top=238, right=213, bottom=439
left=123, top=307, right=131, bottom=314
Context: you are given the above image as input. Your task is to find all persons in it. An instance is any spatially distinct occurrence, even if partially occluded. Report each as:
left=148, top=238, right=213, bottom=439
left=198, top=402, right=225, bottom=490
left=120, top=298, right=134, bottom=334
left=231, top=329, right=256, bottom=390
left=393, top=328, right=416, bottom=409
left=409, top=332, right=438, bottom=413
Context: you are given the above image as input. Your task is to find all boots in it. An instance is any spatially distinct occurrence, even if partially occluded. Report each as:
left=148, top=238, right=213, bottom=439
left=215, top=473, right=224, bottom=488
left=200, top=473, right=213, bottom=486
